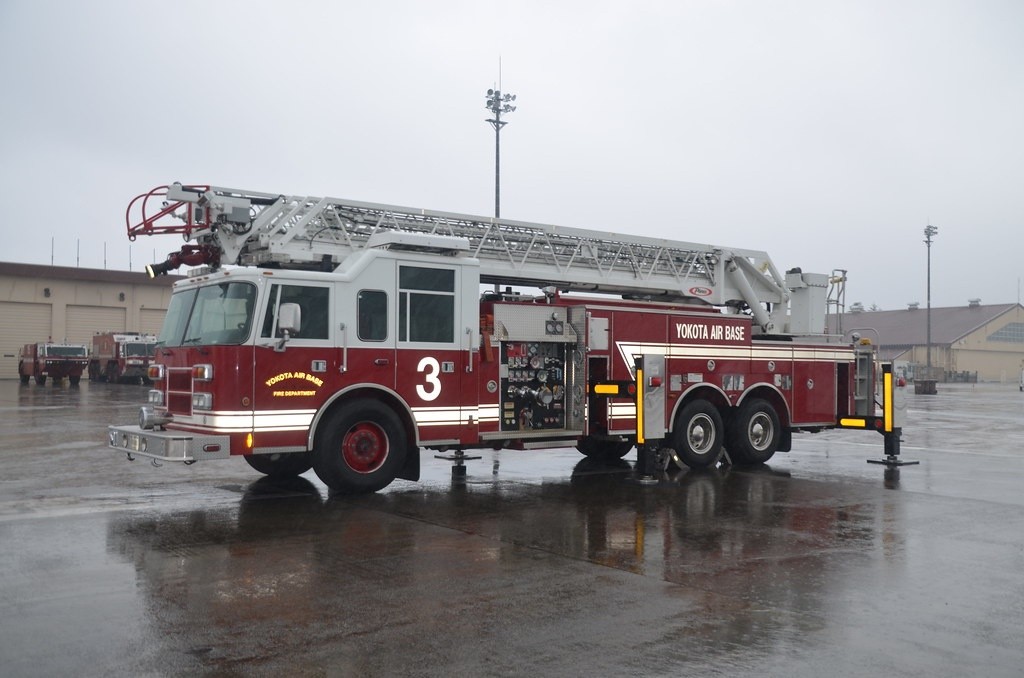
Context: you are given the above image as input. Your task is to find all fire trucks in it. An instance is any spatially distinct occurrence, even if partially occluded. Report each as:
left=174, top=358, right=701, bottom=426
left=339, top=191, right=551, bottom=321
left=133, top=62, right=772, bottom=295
left=108, top=178, right=920, bottom=493
left=87, top=329, right=160, bottom=384
left=16, top=338, right=90, bottom=385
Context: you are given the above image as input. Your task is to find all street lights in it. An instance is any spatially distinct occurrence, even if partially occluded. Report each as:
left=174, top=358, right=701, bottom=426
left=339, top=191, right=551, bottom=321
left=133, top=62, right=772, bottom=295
left=485, top=88, right=517, bottom=293
left=923, top=225, right=939, bottom=378
